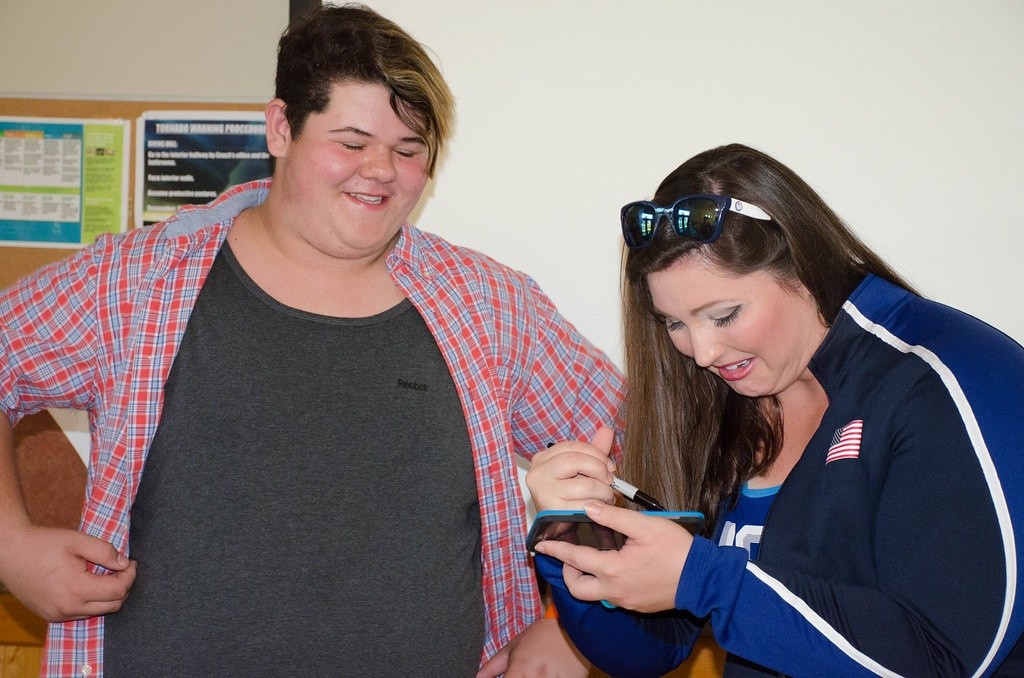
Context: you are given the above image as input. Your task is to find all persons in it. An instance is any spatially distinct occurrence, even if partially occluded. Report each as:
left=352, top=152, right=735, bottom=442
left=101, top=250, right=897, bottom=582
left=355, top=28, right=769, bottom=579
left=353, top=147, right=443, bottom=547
left=525, top=142, right=1024, bottom=678
left=2, top=10, right=628, bottom=678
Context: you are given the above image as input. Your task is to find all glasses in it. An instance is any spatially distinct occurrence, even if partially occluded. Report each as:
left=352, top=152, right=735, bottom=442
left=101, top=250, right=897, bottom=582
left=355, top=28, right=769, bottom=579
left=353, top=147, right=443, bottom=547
left=620, top=198, right=773, bottom=249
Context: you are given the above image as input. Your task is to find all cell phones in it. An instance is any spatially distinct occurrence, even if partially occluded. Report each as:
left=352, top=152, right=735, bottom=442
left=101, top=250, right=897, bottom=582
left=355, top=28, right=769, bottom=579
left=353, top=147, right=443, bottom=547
left=525, top=510, right=704, bottom=555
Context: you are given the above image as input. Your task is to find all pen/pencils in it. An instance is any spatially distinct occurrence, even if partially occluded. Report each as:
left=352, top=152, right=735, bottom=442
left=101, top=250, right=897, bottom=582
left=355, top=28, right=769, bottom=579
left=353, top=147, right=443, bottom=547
left=547, top=442, right=666, bottom=511
